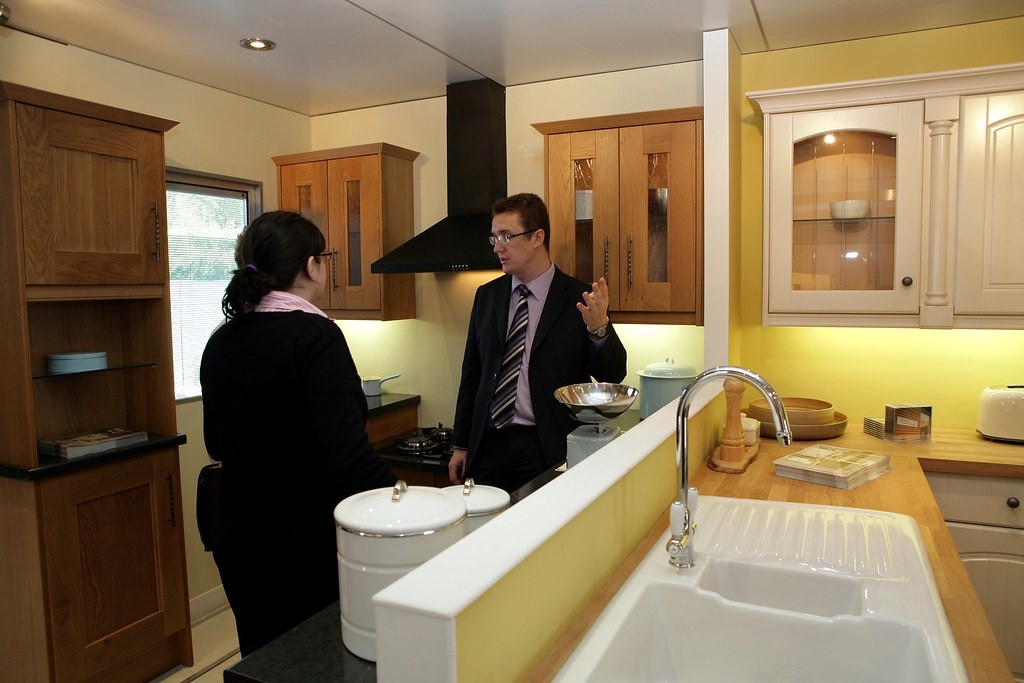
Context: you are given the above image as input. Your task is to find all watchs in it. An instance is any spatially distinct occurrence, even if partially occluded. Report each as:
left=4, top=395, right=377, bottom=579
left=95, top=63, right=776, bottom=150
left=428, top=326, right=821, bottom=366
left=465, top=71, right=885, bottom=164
left=587, top=315, right=609, bottom=337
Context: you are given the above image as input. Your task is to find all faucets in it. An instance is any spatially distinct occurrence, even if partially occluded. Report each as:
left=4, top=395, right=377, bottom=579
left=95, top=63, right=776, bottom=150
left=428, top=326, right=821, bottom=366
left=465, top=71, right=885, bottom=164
left=676, top=366, right=792, bottom=568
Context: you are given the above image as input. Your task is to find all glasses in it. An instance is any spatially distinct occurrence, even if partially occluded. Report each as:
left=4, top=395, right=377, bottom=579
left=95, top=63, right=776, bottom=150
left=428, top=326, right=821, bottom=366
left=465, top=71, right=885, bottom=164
left=314, top=251, right=332, bottom=261
left=489, top=228, right=538, bottom=245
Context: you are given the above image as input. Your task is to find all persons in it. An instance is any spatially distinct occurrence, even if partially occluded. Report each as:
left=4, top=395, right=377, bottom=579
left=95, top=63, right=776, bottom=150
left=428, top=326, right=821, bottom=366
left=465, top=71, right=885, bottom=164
left=448, top=192, right=627, bottom=494
left=196, top=211, right=403, bottom=662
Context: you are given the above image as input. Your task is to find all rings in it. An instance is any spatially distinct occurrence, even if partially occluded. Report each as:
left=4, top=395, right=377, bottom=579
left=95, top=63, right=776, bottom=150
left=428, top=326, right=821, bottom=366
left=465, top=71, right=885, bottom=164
left=589, top=306, right=597, bottom=312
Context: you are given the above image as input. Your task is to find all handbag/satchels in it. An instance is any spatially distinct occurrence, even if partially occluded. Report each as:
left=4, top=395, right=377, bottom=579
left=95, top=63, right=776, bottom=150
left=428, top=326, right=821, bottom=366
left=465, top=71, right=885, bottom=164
left=196, top=464, right=227, bottom=552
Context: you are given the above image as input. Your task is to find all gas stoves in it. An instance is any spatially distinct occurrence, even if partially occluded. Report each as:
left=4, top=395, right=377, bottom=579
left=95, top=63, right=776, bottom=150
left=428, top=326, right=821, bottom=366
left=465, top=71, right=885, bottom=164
left=371, top=422, right=466, bottom=488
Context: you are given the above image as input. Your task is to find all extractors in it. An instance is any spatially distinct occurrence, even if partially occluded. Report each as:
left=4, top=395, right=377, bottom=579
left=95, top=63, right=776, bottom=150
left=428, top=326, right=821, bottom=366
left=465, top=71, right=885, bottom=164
left=370, top=78, right=508, bottom=273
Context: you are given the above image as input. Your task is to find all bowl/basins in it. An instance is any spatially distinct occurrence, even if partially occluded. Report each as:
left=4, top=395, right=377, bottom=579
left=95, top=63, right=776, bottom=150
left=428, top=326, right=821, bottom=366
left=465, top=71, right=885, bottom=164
left=47, top=352, right=108, bottom=373
left=830, top=200, right=870, bottom=218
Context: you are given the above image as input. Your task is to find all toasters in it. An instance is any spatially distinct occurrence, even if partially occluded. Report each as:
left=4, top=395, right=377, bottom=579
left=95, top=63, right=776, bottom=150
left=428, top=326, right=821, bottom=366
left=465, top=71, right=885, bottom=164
left=976, top=383, right=1024, bottom=443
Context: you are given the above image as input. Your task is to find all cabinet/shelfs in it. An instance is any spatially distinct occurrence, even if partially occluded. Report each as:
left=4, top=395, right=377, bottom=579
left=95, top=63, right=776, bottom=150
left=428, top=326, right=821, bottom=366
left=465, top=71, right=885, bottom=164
left=0, top=80, right=194, bottom=683
left=743, top=62, right=1024, bottom=330
left=531, top=104, right=704, bottom=325
left=271, top=141, right=420, bottom=321
left=924, top=470, right=1024, bottom=683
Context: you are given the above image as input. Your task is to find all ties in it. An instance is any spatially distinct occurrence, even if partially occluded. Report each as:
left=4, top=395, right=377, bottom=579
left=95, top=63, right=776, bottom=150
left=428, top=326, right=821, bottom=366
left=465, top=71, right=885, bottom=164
left=490, top=285, right=532, bottom=429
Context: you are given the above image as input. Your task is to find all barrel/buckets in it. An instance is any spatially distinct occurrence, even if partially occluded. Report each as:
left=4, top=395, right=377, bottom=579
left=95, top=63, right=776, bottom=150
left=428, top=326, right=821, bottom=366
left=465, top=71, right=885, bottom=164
left=635, top=358, right=700, bottom=419
left=333, top=480, right=469, bottom=662
left=441, top=477, right=511, bottom=537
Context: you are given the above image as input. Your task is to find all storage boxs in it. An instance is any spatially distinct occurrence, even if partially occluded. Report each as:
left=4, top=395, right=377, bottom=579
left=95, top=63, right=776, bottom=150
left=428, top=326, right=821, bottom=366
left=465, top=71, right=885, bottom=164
left=863, top=403, right=932, bottom=445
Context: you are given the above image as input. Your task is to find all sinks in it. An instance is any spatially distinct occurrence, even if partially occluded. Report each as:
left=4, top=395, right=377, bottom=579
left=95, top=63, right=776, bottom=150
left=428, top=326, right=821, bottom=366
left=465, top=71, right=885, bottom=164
left=696, top=558, right=870, bottom=617
left=583, top=581, right=934, bottom=683
